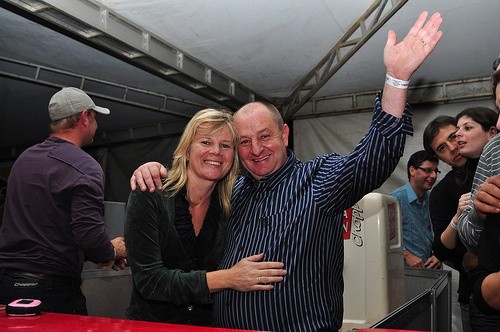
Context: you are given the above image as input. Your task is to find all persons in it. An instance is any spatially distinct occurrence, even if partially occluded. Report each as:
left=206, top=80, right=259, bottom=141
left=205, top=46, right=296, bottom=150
left=423, top=116, right=473, bottom=332
left=468, top=69, right=500, bottom=332
left=123, top=108, right=288, bottom=326
left=0, top=86, right=127, bottom=316
left=432, top=106, right=500, bottom=332
left=386, top=150, right=443, bottom=270
left=130, top=11, right=443, bottom=332
left=456, top=50, right=500, bottom=332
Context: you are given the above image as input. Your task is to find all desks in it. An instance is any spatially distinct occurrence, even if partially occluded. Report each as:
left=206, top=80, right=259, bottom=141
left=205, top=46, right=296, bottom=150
left=0, top=305, right=269, bottom=332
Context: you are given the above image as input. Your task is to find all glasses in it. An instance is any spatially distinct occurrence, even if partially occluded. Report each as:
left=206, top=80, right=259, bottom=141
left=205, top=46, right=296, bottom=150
left=414, top=165, right=441, bottom=174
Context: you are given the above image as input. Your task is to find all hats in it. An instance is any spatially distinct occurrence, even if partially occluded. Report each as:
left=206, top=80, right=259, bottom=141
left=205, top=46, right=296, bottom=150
left=48, top=87, right=111, bottom=122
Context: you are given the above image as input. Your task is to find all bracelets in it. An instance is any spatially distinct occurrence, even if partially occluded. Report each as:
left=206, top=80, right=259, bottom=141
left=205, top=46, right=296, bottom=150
left=111, top=246, right=116, bottom=260
left=385, top=74, right=411, bottom=89
left=450, top=218, right=458, bottom=231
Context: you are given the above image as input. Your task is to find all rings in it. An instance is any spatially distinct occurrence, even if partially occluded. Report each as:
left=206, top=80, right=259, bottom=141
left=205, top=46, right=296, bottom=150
left=421, top=40, right=426, bottom=46
left=258, top=277, right=261, bottom=283
